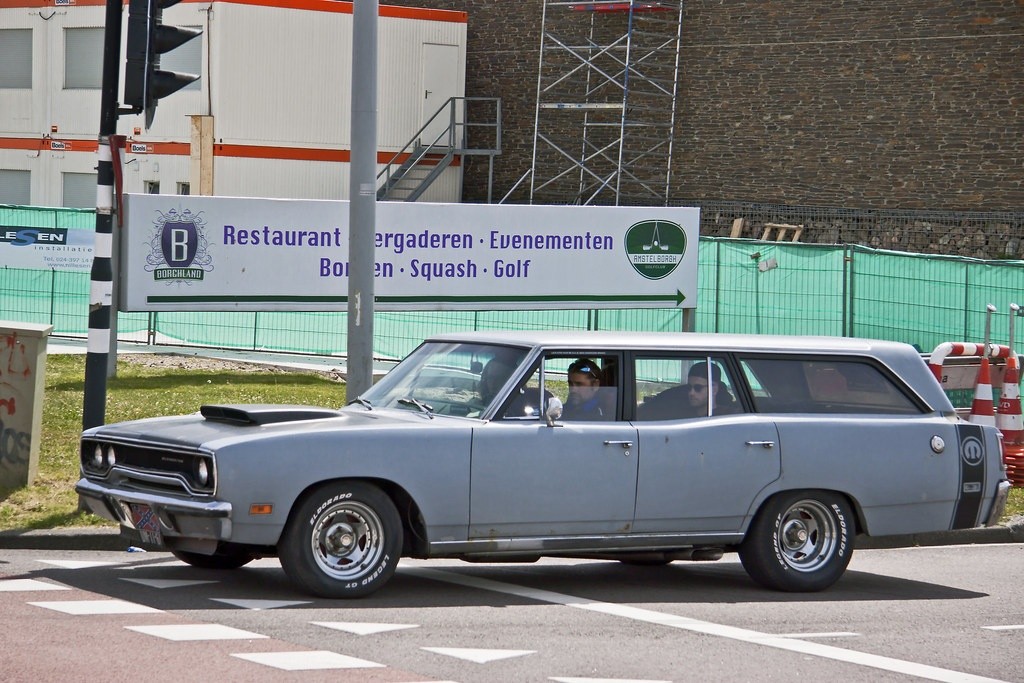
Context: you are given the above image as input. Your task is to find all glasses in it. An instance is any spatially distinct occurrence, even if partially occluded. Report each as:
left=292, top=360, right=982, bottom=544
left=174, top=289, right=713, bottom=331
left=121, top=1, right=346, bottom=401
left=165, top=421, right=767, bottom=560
left=568, top=363, right=597, bottom=379
left=683, top=382, right=714, bottom=393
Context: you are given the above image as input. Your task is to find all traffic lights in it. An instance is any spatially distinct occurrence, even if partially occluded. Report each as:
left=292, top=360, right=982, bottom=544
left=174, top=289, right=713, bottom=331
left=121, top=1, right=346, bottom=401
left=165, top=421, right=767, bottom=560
left=123, top=0, right=202, bottom=133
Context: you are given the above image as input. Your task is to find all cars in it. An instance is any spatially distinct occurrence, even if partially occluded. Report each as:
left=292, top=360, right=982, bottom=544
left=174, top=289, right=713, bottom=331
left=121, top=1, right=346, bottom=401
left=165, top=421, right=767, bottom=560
left=73, top=328, right=1011, bottom=600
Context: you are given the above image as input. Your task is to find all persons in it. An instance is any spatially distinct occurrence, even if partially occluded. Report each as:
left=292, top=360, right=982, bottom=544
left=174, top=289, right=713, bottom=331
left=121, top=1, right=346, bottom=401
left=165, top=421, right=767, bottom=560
left=560, top=358, right=609, bottom=419
left=679, top=362, right=734, bottom=416
left=476, top=357, right=534, bottom=417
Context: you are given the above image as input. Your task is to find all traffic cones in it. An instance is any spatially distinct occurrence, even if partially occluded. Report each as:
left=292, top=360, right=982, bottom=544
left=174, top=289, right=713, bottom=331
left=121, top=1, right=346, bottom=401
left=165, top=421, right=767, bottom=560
left=968, top=357, right=994, bottom=428
left=999, top=356, right=1024, bottom=486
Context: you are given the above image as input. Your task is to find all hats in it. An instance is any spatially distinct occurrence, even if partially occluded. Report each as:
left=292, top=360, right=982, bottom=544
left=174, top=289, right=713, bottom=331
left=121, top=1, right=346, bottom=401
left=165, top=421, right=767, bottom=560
left=689, top=362, right=721, bottom=381
